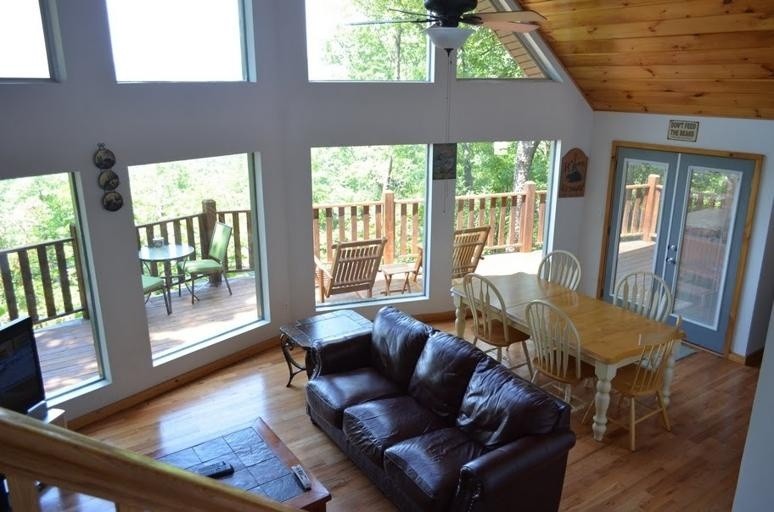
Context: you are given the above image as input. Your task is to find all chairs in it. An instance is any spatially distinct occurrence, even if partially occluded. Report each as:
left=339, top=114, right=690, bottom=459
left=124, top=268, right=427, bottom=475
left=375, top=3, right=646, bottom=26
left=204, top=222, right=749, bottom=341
left=178, top=222, right=232, bottom=304
left=536, top=250, right=582, bottom=291
left=313, top=235, right=387, bottom=303
left=523, top=299, right=593, bottom=414
left=412, top=225, right=492, bottom=283
left=462, top=273, right=530, bottom=377
left=612, top=272, right=671, bottom=324
left=581, top=318, right=686, bottom=450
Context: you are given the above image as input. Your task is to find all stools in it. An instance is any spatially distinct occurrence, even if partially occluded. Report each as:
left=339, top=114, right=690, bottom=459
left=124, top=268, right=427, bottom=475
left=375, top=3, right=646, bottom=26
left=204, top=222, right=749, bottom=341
left=142, top=273, right=171, bottom=315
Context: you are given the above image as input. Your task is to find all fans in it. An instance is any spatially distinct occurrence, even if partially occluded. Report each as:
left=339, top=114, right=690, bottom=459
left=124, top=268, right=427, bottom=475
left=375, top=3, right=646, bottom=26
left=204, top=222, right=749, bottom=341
left=350, top=2, right=547, bottom=31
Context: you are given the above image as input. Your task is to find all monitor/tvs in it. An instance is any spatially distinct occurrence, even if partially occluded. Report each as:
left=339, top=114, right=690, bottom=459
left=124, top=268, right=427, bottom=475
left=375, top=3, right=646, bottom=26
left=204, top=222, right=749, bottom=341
left=0, top=313, right=47, bottom=416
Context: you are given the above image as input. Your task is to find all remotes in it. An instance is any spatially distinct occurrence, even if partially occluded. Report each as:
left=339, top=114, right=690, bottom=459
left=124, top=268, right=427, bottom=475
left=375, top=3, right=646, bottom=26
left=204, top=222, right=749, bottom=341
left=199, top=463, right=233, bottom=477
left=290, top=462, right=314, bottom=491
left=197, top=461, right=226, bottom=472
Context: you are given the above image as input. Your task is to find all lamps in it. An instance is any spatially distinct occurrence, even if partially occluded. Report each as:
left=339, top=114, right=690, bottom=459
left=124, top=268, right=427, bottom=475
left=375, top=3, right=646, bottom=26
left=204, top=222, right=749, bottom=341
left=423, top=23, right=475, bottom=58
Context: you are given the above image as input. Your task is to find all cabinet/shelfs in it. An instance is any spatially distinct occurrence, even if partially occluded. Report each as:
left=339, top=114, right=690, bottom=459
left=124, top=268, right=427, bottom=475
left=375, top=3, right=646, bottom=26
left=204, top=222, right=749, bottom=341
left=29, top=408, right=67, bottom=429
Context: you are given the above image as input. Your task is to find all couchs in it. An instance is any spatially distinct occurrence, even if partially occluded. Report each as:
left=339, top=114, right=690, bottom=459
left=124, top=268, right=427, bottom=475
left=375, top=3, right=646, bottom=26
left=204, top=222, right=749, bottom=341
left=305, top=307, right=576, bottom=512
